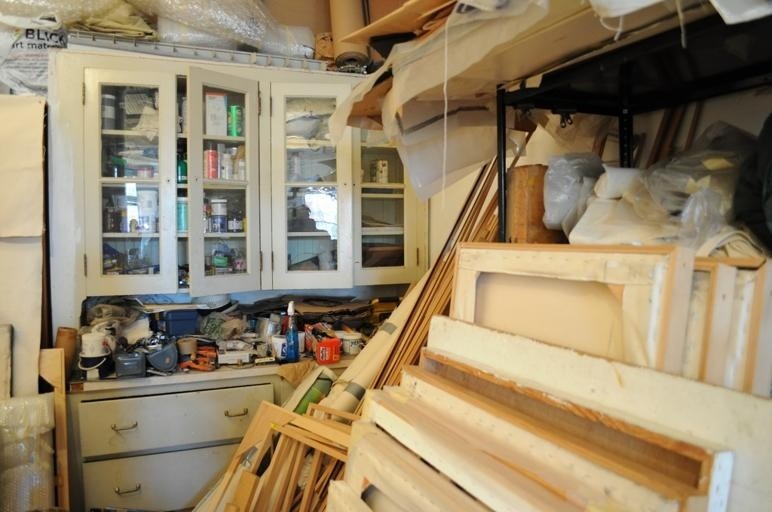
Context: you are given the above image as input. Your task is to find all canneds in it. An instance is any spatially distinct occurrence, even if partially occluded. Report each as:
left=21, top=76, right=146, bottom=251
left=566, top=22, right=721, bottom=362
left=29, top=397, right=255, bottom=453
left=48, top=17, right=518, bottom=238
left=226, top=147, right=238, bottom=156
left=370, top=159, right=388, bottom=183
left=102, top=93, right=117, bottom=130
left=227, top=104, right=243, bottom=137
left=203, top=149, right=218, bottom=180
left=210, top=198, right=243, bottom=232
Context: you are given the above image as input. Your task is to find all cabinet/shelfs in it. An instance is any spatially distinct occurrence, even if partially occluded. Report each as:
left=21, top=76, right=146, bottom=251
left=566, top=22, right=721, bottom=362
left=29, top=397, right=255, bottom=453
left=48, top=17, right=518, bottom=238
left=80, top=65, right=263, bottom=298
left=71, top=375, right=275, bottom=511
left=270, top=80, right=419, bottom=290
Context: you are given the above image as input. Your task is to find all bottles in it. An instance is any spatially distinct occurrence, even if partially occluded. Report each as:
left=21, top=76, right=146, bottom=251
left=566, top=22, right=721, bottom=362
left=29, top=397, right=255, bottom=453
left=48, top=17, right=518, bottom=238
left=178, top=154, right=187, bottom=184
left=234, top=143, right=245, bottom=181
left=105, top=197, right=118, bottom=231
left=119, top=195, right=140, bottom=231
left=285, top=300, right=298, bottom=361
left=101, top=94, right=116, bottom=130
left=221, top=151, right=233, bottom=179
left=290, top=152, right=303, bottom=179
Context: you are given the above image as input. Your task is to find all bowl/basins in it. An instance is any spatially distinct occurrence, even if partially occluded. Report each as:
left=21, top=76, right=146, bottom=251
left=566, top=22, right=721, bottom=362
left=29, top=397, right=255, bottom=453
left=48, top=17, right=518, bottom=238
left=287, top=116, right=320, bottom=140
left=364, top=243, right=403, bottom=265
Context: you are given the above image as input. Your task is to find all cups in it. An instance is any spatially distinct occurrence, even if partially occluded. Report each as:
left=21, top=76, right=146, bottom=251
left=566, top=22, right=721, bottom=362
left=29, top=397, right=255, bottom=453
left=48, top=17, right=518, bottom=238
left=343, top=333, right=365, bottom=354
left=82, top=332, right=107, bottom=354
left=270, top=334, right=287, bottom=360
left=298, top=331, right=305, bottom=352
left=176, top=197, right=189, bottom=231
left=178, top=338, right=198, bottom=361
left=109, top=166, right=123, bottom=177
left=369, top=158, right=388, bottom=181
